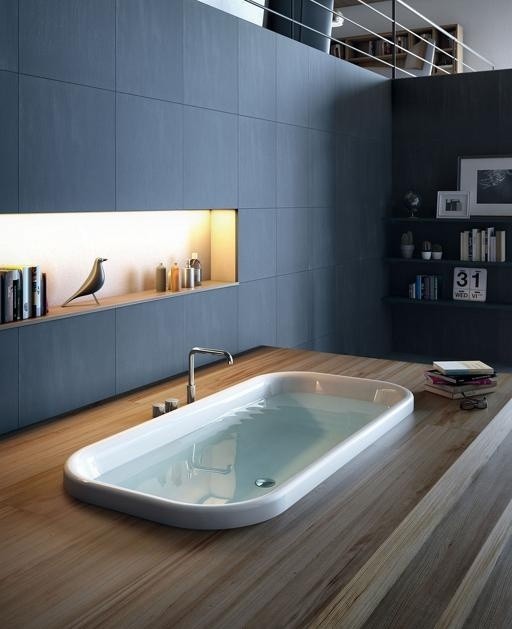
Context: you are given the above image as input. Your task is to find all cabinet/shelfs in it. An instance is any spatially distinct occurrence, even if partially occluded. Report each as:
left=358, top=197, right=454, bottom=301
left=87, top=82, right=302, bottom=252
left=330, top=22, right=465, bottom=76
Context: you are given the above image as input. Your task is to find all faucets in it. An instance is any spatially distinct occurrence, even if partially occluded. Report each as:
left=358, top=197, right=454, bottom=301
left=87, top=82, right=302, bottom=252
left=187, top=347, right=234, bottom=404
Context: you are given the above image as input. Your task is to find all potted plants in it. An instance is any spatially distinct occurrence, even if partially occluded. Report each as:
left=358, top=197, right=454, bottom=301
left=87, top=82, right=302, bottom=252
left=400, top=231, right=415, bottom=259
left=420, top=240, right=431, bottom=260
left=432, top=244, right=443, bottom=260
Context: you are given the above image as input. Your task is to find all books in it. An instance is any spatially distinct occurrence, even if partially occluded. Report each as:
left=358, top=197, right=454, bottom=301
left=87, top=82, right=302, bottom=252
left=0, top=265, right=49, bottom=324
left=329, top=44, right=345, bottom=59
left=368, top=32, right=432, bottom=56
left=423, top=360, right=497, bottom=400
left=460, top=227, right=506, bottom=263
left=408, top=275, right=438, bottom=300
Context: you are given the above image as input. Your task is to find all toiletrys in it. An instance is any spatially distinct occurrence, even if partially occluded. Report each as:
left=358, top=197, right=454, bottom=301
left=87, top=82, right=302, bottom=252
left=155, top=251, right=202, bottom=292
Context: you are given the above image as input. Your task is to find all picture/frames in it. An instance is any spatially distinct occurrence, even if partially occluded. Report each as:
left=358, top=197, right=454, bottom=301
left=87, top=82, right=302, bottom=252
left=456, top=154, right=512, bottom=218
left=435, top=190, right=471, bottom=220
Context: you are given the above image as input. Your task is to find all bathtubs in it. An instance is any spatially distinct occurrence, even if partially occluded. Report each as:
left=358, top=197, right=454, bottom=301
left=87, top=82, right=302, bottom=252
left=62, top=370, right=414, bottom=530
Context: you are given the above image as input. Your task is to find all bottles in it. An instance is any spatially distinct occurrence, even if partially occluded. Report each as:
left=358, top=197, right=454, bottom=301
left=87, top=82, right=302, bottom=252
left=156, top=262, right=167, bottom=292
left=184, top=258, right=195, bottom=288
left=170, top=261, right=180, bottom=291
left=188, top=252, right=203, bottom=286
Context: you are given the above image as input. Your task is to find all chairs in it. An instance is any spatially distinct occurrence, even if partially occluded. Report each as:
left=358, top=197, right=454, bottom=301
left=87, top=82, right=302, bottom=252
left=359, top=37, right=437, bottom=78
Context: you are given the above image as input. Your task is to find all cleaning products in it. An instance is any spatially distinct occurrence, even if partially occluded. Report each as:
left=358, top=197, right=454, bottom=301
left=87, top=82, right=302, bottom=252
left=155, top=252, right=202, bottom=292
